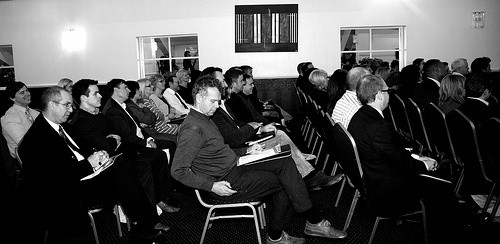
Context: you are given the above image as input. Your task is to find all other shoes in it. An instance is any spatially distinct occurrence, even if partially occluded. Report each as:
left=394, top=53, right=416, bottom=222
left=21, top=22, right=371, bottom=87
left=302, top=153, right=317, bottom=160
left=310, top=171, right=343, bottom=191
left=266, top=230, right=305, bottom=244
left=304, top=219, right=348, bottom=238
left=154, top=222, right=171, bottom=234
left=155, top=200, right=181, bottom=216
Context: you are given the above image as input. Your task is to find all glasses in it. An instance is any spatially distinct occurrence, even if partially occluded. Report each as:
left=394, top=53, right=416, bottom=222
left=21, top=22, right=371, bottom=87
left=380, top=88, right=396, bottom=95
left=156, top=79, right=165, bottom=83
left=66, top=84, right=73, bottom=88
left=146, top=84, right=153, bottom=87
left=174, top=81, right=179, bottom=84
left=52, top=100, right=73, bottom=108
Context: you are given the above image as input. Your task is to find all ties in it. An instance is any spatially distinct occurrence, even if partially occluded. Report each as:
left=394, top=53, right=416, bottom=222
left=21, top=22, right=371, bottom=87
left=25, top=110, right=35, bottom=124
left=59, top=125, right=87, bottom=159
left=175, top=93, right=188, bottom=109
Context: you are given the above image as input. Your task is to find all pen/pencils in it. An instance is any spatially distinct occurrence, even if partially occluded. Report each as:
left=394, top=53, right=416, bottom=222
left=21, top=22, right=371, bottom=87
left=259, top=121, right=264, bottom=125
left=92, top=147, right=98, bottom=152
left=246, top=143, right=266, bottom=156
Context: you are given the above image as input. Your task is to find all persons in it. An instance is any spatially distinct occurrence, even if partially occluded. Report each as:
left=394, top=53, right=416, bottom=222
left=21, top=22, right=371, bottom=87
left=0, top=47, right=500, bottom=244
left=171, top=75, right=347, bottom=244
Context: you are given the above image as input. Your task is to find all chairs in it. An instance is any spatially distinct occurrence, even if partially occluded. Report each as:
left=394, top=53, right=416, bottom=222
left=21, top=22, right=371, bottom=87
left=18, top=83, right=500, bottom=244
